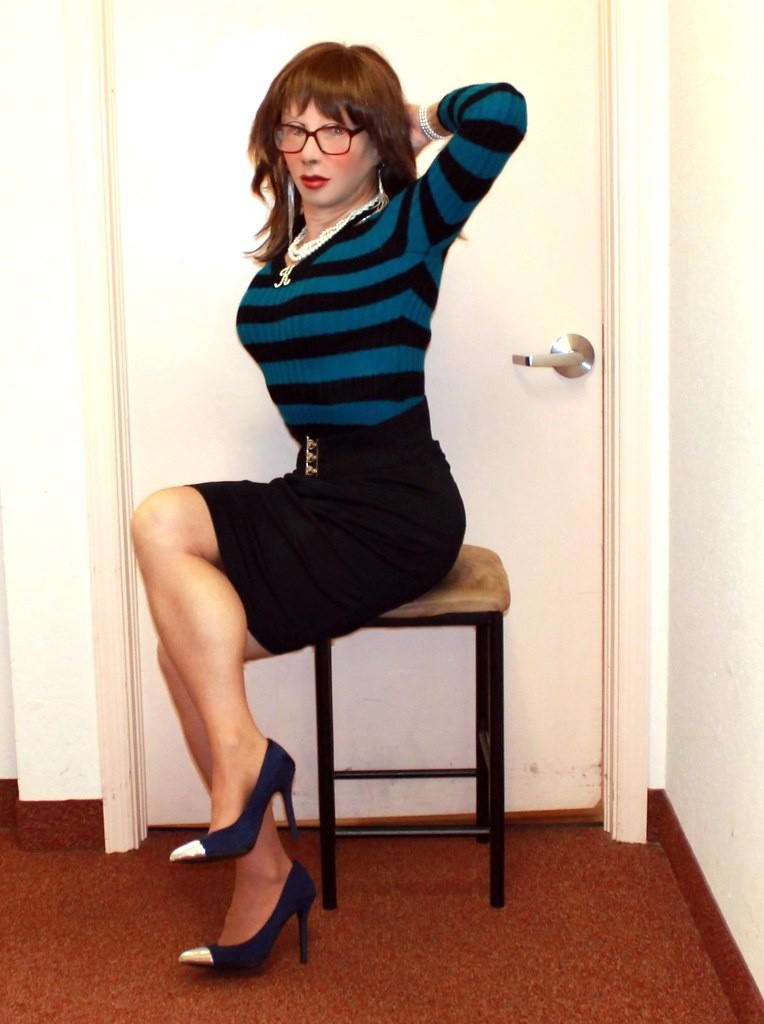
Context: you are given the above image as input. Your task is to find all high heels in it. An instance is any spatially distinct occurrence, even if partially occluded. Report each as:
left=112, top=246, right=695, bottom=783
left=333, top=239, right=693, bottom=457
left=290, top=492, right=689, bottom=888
left=170, top=739, right=297, bottom=864
left=178, top=859, right=317, bottom=970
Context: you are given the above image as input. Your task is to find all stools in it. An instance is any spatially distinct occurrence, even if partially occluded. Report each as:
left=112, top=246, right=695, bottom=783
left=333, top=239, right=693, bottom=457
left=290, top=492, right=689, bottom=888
left=314, top=544, right=511, bottom=911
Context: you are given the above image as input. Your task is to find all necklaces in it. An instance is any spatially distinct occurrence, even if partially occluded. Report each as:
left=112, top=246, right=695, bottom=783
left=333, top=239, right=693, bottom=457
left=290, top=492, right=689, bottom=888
left=288, top=193, right=380, bottom=262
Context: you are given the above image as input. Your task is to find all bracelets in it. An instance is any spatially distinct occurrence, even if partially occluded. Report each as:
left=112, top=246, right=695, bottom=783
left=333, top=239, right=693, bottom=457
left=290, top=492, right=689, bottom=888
left=417, top=104, right=453, bottom=141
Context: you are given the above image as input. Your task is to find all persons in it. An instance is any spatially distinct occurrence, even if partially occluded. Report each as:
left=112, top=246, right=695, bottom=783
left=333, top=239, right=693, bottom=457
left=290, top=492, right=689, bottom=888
left=131, top=43, right=527, bottom=969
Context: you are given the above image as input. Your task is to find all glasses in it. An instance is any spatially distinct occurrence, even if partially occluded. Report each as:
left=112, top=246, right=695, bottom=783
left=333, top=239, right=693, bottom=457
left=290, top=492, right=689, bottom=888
left=274, top=123, right=367, bottom=155
